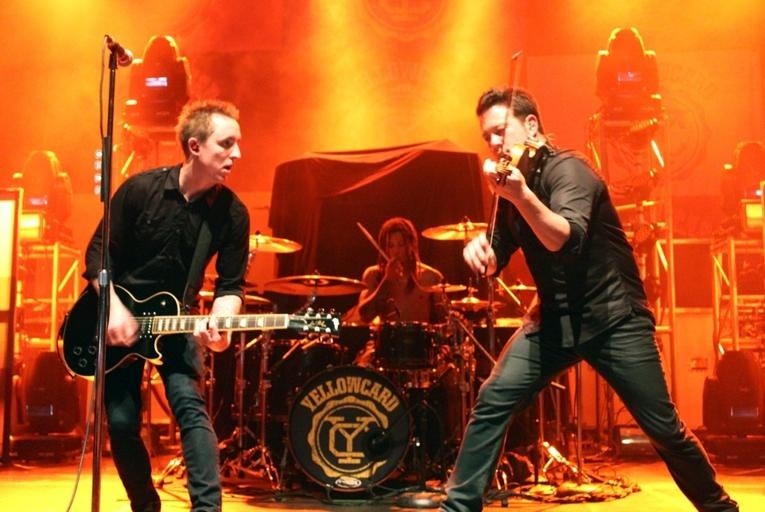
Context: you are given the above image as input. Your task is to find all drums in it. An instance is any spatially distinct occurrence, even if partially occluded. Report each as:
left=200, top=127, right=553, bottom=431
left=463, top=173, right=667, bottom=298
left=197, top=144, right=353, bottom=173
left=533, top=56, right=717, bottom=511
left=373, top=321, right=434, bottom=374
left=285, top=365, right=412, bottom=494
left=337, top=323, right=371, bottom=367
left=473, top=318, right=524, bottom=383
left=230, top=331, right=346, bottom=422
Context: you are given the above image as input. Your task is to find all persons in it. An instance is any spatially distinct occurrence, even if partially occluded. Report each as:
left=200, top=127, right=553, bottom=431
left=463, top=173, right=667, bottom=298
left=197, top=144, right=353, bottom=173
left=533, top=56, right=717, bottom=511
left=356, top=218, right=467, bottom=450
left=431, top=85, right=739, bottom=512
left=82, top=95, right=251, bottom=512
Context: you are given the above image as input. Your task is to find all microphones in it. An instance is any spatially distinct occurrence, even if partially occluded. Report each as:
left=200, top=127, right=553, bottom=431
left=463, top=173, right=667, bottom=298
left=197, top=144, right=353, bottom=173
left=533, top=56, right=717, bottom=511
left=104, top=32, right=133, bottom=67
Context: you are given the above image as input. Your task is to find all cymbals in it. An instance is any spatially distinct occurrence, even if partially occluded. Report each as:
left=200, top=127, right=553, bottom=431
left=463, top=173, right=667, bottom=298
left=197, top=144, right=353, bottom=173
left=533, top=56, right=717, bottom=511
left=421, top=222, right=492, bottom=241
left=263, top=276, right=369, bottom=297
left=248, top=235, right=304, bottom=253
left=496, top=283, right=536, bottom=292
left=197, top=291, right=270, bottom=306
left=449, top=297, right=501, bottom=311
left=424, top=284, right=477, bottom=294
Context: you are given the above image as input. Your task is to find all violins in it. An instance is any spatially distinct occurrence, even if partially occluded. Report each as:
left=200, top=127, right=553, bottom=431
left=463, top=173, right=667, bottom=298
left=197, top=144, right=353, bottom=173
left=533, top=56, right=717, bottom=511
left=494, top=141, right=550, bottom=239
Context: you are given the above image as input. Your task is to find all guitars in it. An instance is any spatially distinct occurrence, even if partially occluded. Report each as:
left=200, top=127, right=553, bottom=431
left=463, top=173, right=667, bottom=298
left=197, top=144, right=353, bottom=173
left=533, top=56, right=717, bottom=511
left=61, top=285, right=343, bottom=381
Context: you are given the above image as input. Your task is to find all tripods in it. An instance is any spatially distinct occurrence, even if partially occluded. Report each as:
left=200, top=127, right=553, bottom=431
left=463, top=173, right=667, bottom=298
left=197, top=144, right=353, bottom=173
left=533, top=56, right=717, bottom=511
left=156, top=302, right=282, bottom=494
left=461, top=311, right=584, bottom=505
